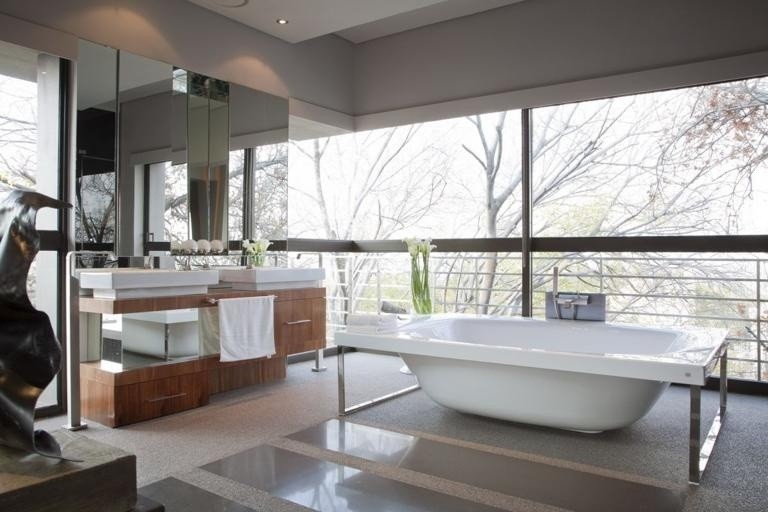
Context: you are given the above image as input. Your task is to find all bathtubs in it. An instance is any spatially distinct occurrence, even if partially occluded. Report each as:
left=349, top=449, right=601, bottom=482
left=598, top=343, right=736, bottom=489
left=335, top=312, right=727, bottom=435
left=123, top=307, right=199, bottom=360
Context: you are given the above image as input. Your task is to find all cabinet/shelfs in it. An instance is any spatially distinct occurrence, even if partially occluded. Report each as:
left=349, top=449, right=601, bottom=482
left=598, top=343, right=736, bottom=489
left=79, top=288, right=329, bottom=428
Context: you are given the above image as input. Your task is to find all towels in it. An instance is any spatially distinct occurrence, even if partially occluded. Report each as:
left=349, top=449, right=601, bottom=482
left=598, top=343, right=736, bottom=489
left=215, top=295, right=280, bottom=365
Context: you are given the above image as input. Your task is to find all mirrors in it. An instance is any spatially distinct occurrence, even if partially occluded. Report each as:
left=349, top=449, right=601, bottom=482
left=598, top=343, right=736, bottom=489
left=80, top=307, right=221, bottom=372
left=199, top=309, right=220, bottom=358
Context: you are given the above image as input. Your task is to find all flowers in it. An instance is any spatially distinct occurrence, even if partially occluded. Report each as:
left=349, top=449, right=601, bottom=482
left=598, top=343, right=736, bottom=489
left=402, top=235, right=435, bottom=313
left=243, top=238, right=273, bottom=265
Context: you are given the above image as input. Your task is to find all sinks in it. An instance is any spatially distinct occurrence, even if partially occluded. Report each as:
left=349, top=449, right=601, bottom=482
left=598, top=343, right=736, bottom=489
left=219, top=266, right=326, bottom=290
left=79, top=269, right=218, bottom=300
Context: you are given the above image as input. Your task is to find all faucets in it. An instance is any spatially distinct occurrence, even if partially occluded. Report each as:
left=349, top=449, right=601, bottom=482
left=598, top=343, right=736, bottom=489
left=563, top=298, right=576, bottom=319
left=246, top=255, right=255, bottom=269
left=177, top=259, right=191, bottom=270
